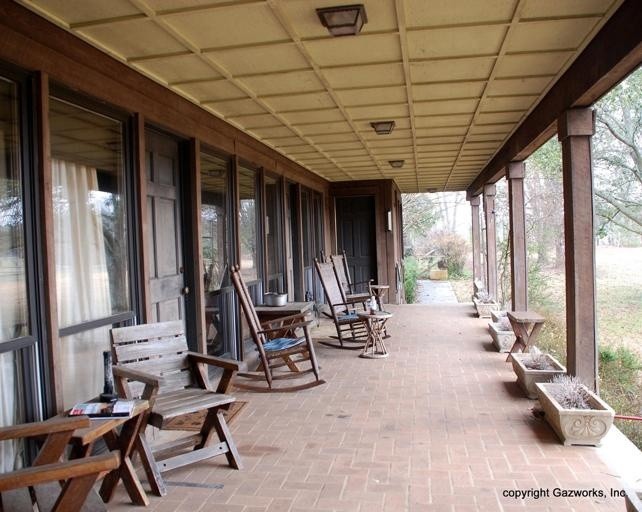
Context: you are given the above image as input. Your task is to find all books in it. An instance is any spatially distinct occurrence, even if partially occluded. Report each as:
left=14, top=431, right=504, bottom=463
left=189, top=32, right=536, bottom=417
left=69, top=400, right=136, bottom=417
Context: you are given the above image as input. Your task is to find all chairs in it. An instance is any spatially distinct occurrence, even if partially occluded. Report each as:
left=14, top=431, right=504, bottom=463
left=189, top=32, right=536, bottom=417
left=230, top=263, right=327, bottom=393
left=313, top=254, right=391, bottom=350
left=0, top=415, right=119, bottom=512
left=109, top=319, right=247, bottom=497
left=330, top=250, right=379, bottom=311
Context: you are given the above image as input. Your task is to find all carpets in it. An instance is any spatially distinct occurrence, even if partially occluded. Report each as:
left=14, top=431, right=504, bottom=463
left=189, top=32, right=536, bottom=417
left=161, top=399, right=249, bottom=432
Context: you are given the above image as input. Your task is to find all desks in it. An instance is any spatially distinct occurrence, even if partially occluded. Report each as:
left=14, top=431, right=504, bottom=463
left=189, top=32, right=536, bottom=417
left=357, top=311, right=392, bottom=360
left=252, top=301, right=315, bottom=372
left=41, top=394, right=149, bottom=505
left=506, top=311, right=546, bottom=362
left=366, top=284, right=389, bottom=311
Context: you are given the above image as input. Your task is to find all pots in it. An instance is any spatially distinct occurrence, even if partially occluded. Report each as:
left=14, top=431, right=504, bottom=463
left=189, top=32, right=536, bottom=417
left=263, top=292, right=288, bottom=308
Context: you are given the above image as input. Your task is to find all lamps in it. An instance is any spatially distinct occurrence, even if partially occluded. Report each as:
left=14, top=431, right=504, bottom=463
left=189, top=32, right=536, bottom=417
left=315, top=4, right=368, bottom=38
left=389, top=160, right=404, bottom=168
left=370, top=121, right=396, bottom=135
